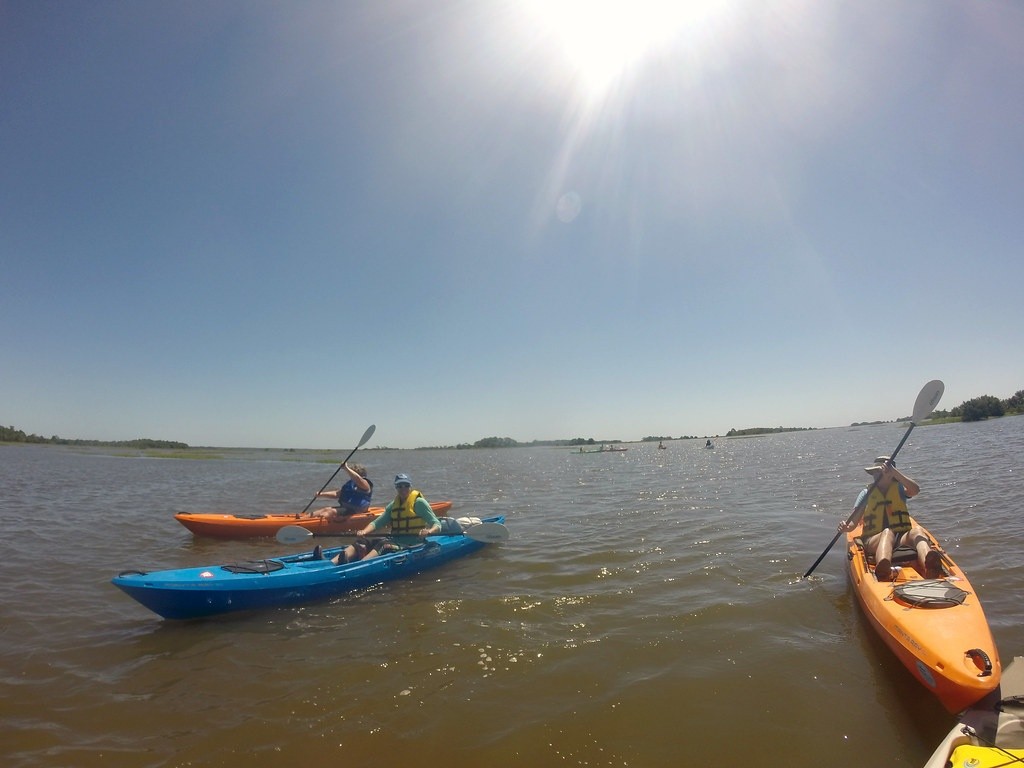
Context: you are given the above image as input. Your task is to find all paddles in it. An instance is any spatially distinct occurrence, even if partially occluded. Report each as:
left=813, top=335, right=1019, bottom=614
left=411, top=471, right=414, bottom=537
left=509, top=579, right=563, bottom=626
left=275, top=522, right=511, bottom=546
left=803, top=380, right=945, bottom=582
left=301, top=424, right=376, bottom=514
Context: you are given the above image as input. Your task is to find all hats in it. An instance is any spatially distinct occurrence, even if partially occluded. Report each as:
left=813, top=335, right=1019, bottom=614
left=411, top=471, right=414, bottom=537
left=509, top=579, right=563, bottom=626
left=864, top=456, right=896, bottom=475
left=393, top=474, right=410, bottom=485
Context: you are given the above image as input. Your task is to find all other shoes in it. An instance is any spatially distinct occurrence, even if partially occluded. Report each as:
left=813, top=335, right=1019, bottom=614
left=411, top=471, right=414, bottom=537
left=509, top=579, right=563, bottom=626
left=310, top=512, right=314, bottom=518
left=876, top=556, right=892, bottom=583
left=924, top=550, right=941, bottom=579
left=338, top=551, right=349, bottom=566
left=296, top=514, right=300, bottom=519
left=313, top=545, right=325, bottom=560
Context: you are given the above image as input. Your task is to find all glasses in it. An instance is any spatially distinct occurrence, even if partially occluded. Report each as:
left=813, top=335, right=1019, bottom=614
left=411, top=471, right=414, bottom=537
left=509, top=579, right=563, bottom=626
left=394, top=482, right=411, bottom=488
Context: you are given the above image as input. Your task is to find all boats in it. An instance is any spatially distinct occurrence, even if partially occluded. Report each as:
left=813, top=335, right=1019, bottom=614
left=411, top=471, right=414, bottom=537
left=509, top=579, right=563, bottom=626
left=845, top=511, right=1002, bottom=716
left=173, top=499, right=456, bottom=540
left=919, top=655, right=1024, bottom=768
left=112, top=512, right=510, bottom=623
left=571, top=447, right=629, bottom=454
left=704, top=444, right=715, bottom=449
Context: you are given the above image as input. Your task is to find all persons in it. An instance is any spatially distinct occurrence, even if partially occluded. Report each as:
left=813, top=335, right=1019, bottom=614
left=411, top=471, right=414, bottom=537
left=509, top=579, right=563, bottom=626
left=610, top=444, right=613, bottom=450
left=304, top=464, right=374, bottom=519
left=706, top=440, right=711, bottom=446
left=659, top=442, right=663, bottom=448
left=331, top=474, right=443, bottom=565
left=836, top=456, right=942, bottom=581
left=580, top=447, right=583, bottom=452
